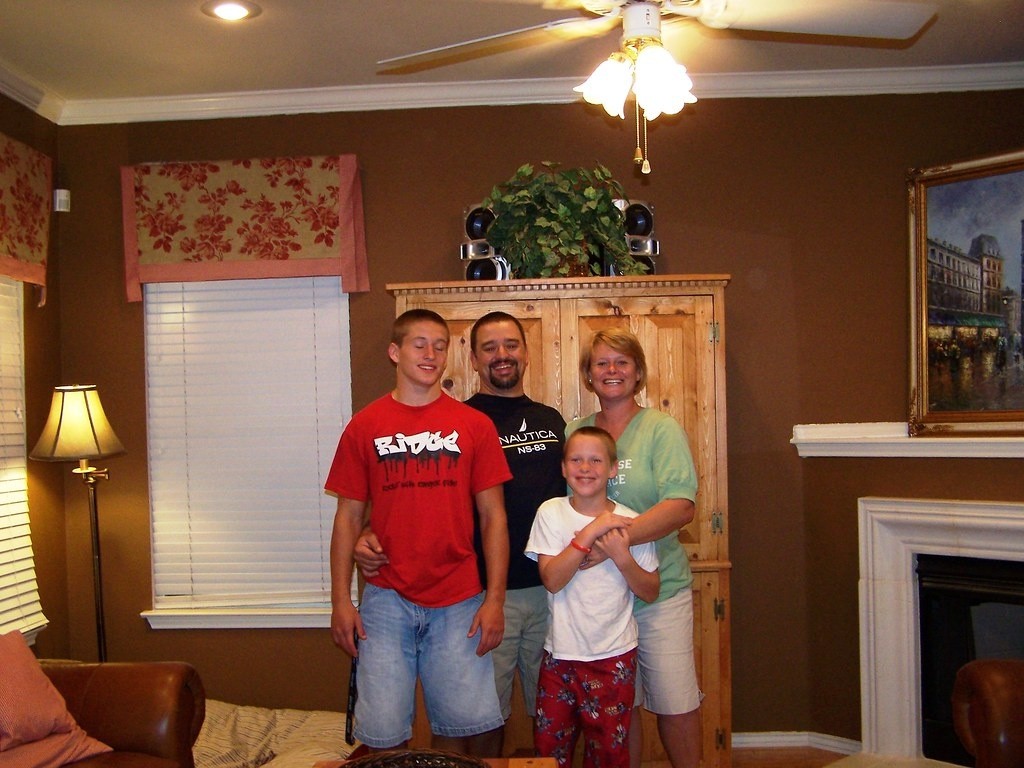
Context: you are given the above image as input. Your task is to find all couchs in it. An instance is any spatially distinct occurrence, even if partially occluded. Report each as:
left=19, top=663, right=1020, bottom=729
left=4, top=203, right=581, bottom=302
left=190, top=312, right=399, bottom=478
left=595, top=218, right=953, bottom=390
left=23, top=656, right=207, bottom=768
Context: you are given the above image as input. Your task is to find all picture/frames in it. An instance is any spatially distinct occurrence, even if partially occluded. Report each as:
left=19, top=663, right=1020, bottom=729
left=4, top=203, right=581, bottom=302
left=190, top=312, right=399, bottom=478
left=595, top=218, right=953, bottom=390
left=905, top=151, right=1024, bottom=439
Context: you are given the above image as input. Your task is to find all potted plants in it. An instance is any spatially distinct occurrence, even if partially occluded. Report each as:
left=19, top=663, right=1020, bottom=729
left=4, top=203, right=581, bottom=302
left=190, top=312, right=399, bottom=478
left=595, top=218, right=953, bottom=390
left=478, top=159, right=654, bottom=278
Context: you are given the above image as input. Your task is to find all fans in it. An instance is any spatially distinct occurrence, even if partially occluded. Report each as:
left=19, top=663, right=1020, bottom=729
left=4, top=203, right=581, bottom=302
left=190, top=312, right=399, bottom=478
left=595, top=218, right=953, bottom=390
left=376, top=0, right=939, bottom=74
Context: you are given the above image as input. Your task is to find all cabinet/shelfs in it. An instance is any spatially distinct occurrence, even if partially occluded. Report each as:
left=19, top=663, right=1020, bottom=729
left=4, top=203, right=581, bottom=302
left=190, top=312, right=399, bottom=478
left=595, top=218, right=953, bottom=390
left=383, top=274, right=732, bottom=768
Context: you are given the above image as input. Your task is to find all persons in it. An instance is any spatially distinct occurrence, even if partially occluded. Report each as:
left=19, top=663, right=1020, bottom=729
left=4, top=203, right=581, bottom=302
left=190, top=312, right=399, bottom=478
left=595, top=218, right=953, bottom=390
left=324, top=307, right=512, bottom=761
left=554, top=326, right=702, bottom=768
left=355, top=310, right=565, bottom=754
left=525, top=424, right=660, bottom=768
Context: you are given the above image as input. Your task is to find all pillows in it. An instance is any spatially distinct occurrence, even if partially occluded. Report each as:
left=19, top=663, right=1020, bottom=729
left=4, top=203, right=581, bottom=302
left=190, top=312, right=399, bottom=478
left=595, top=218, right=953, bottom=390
left=0, top=630, right=115, bottom=768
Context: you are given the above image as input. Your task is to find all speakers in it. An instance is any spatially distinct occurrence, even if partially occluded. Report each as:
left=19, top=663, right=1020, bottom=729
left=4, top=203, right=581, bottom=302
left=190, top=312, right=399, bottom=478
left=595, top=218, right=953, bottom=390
left=587, top=198, right=659, bottom=277
left=460, top=203, right=512, bottom=281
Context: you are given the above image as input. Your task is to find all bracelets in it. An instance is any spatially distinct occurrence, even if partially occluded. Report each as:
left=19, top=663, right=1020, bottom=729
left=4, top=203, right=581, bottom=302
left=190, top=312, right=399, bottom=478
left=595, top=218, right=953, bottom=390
left=569, top=540, right=591, bottom=553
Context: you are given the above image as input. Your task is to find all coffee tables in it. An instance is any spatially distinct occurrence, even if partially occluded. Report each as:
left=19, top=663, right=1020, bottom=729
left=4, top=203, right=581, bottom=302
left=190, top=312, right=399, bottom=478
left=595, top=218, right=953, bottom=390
left=313, top=757, right=559, bottom=768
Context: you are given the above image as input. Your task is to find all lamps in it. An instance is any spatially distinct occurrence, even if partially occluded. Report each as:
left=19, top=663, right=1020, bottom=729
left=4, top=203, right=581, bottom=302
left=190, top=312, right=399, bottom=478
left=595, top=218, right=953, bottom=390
left=26, top=384, right=130, bottom=661
left=571, top=41, right=699, bottom=172
